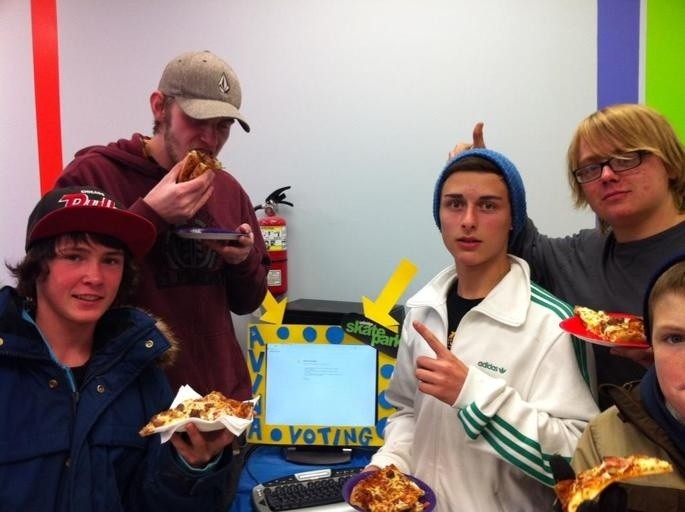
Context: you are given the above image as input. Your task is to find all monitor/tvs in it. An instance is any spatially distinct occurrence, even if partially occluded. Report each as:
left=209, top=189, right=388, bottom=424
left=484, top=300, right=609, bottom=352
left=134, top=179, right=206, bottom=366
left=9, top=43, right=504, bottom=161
left=248, top=324, right=399, bottom=464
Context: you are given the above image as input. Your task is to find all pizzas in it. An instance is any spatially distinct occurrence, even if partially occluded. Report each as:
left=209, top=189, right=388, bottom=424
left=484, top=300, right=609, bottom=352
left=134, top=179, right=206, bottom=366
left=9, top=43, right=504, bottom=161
left=574, top=305, right=647, bottom=345
left=348, top=463, right=426, bottom=512
left=555, top=455, right=674, bottom=512
left=177, top=149, right=224, bottom=183
left=138, top=389, right=254, bottom=439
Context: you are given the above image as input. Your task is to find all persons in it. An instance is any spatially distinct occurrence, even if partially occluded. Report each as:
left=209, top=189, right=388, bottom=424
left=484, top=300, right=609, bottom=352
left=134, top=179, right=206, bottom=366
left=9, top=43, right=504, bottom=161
left=448, top=102, right=685, bottom=413
left=548, top=247, right=685, bottom=512
left=0, top=184, right=258, bottom=511
left=360, top=147, right=603, bottom=512
left=53, top=49, right=272, bottom=511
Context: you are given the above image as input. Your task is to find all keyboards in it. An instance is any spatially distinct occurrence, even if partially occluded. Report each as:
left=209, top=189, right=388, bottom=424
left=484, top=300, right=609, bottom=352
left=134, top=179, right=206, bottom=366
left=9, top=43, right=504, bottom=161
left=249, top=466, right=373, bottom=507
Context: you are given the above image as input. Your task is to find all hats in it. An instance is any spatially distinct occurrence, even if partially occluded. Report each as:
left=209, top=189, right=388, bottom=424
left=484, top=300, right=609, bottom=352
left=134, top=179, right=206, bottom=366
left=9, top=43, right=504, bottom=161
left=433, top=148, right=526, bottom=249
left=25, top=185, right=158, bottom=264
left=158, top=49, right=251, bottom=132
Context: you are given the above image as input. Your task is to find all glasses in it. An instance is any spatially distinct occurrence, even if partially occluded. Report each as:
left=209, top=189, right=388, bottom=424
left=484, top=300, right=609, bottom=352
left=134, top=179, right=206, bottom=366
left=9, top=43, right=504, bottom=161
left=572, top=149, right=652, bottom=184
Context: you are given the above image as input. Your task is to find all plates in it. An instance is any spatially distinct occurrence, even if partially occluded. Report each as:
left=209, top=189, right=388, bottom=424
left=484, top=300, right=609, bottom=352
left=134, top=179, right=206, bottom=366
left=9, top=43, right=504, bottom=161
left=559, top=311, right=650, bottom=349
left=342, top=471, right=436, bottom=512
left=174, top=227, right=247, bottom=241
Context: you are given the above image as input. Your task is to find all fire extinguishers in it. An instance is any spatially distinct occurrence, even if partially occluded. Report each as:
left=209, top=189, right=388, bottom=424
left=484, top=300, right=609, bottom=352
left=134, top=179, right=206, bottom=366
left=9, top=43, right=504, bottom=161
left=254, top=186, right=294, bottom=296
